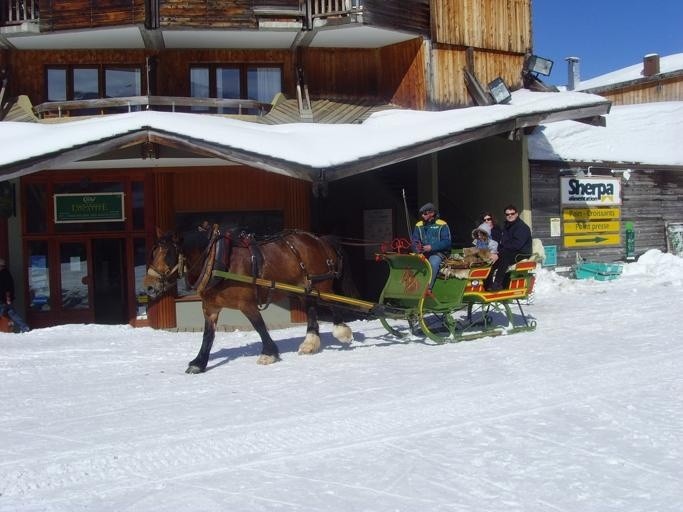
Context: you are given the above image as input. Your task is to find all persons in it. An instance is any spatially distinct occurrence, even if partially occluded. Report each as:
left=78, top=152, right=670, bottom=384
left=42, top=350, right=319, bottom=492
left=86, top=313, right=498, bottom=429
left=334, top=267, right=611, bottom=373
left=471, top=221, right=498, bottom=255
left=404, top=203, right=452, bottom=293
left=477, top=211, right=500, bottom=239
left=483, top=204, right=532, bottom=291
left=1, top=258, right=32, bottom=332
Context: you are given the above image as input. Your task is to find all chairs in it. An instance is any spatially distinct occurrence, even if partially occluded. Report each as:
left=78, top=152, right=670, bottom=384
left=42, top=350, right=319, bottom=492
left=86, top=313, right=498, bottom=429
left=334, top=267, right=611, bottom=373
left=433, top=238, right=538, bottom=294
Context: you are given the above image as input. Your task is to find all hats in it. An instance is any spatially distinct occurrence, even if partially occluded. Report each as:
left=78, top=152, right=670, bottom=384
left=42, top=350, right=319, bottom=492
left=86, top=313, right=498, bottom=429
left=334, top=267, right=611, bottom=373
left=418, top=201, right=436, bottom=213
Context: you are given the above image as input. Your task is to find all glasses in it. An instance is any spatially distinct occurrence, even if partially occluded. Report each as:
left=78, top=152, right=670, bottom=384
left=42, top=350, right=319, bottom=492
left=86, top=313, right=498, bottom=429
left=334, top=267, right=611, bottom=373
left=421, top=211, right=433, bottom=215
left=503, top=212, right=516, bottom=216
left=482, top=218, right=491, bottom=221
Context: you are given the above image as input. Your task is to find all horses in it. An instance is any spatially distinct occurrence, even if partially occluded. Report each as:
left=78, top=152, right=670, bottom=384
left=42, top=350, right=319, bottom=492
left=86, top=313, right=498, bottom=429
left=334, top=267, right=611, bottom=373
left=143, top=226, right=362, bottom=375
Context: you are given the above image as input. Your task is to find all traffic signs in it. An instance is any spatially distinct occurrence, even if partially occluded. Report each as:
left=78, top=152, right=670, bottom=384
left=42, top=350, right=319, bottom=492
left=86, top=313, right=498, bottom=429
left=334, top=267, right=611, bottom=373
left=563, top=207, right=621, bottom=245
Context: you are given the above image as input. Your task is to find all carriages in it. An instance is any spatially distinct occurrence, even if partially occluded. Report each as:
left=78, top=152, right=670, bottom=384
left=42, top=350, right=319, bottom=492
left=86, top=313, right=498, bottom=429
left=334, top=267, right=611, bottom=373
left=143, top=213, right=547, bottom=374
